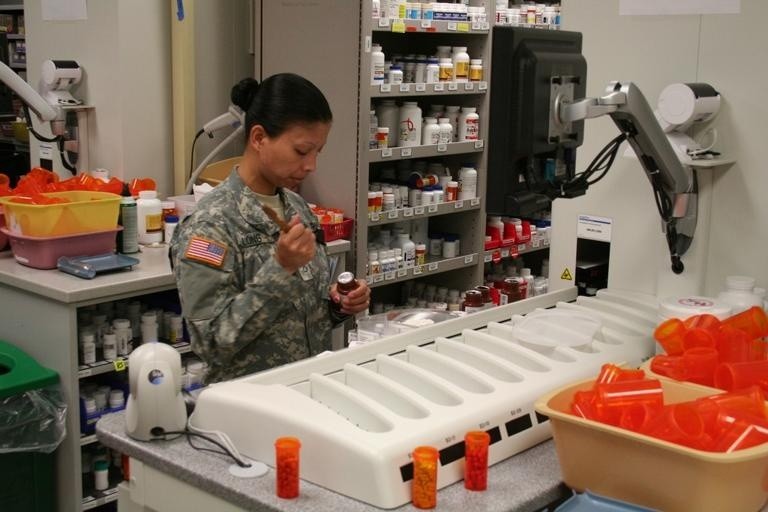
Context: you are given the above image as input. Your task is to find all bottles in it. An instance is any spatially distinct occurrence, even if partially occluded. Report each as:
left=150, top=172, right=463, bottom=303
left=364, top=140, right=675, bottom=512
left=115, top=183, right=178, bottom=255
left=372, top=44, right=484, bottom=86
left=79, top=381, right=124, bottom=420
left=468, top=255, right=554, bottom=311
left=368, top=97, right=480, bottom=150
left=180, top=355, right=208, bottom=389
left=86, top=441, right=122, bottom=490
left=367, top=221, right=461, bottom=274
left=486, top=214, right=550, bottom=241
left=372, top=1, right=487, bottom=21
left=309, top=203, right=345, bottom=234
left=78, top=300, right=185, bottom=364
left=367, top=161, right=479, bottom=215
left=337, top=272, right=359, bottom=309
left=496, top=1, right=560, bottom=24
left=654, top=274, right=768, bottom=322
left=371, top=279, right=467, bottom=315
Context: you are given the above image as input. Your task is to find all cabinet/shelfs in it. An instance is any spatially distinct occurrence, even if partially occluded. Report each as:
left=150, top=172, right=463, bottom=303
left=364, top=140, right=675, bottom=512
left=244, top=1, right=560, bottom=351
left=0, top=238, right=354, bottom=511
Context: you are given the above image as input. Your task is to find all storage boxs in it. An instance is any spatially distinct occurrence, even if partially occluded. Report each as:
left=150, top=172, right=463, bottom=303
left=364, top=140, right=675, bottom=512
left=1, top=225, right=127, bottom=270
left=533, top=369, right=767, bottom=510
left=639, top=354, right=726, bottom=393
left=190, top=153, right=244, bottom=188
left=0, top=191, right=125, bottom=239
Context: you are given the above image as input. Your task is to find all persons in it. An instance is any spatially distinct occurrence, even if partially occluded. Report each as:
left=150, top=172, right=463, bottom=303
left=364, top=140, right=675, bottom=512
left=169, top=74, right=372, bottom=388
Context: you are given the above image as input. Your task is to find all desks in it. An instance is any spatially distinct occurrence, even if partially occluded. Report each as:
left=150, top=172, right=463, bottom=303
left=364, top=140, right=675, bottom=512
left=90, top=366, right=567, bottom=510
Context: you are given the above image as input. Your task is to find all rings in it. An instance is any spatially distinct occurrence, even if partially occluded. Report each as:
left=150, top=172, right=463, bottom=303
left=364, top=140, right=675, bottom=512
left=364, top=299, right=372, bottom=307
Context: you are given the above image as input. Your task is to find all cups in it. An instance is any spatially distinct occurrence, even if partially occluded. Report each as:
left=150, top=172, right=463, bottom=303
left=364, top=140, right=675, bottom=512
left=412, top=446, right=438, bottom=509
left=275, top=436, right=300, bottom=499
left=650, top=306, right=768, bottom=401
left=562, top=361, right=768, bottom=453
left=464, top=431, right=490, bottom=490
left=0, top=168, right=156, bottom=204
